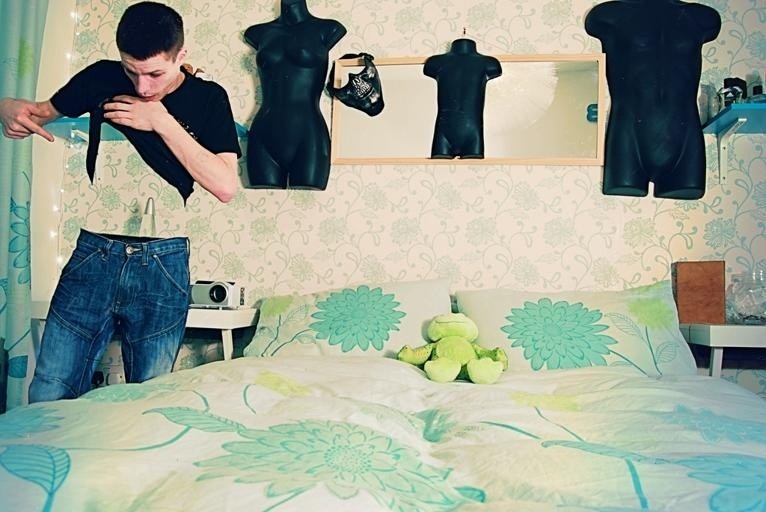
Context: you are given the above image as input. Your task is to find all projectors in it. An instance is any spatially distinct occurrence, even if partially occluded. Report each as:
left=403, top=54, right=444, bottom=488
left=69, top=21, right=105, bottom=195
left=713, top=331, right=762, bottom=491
left=187, top=280, right=245, bottom=310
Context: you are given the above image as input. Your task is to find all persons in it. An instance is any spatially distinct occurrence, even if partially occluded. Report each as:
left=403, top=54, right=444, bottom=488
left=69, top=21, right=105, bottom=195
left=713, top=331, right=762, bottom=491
left=424, top=39, right=502, bottom=160
left=244, top=0, right=346, bottom=190
left=585, top=0, right=721, bottom=199
left=1, top=0, right=242, bottom=405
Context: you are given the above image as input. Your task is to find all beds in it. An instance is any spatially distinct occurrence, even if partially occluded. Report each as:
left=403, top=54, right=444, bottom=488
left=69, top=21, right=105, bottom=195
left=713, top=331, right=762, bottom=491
left=1, top=356, right=765, bottom=511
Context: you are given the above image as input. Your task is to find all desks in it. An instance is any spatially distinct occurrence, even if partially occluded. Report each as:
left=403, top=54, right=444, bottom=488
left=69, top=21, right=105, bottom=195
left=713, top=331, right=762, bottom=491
left=31, top=302, right=259, bottom=361
left=680, top=322, right=766, bottom=376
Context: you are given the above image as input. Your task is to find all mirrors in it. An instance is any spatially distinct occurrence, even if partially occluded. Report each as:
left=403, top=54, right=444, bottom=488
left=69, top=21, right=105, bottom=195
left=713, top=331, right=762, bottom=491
left=330, top=54, right=606, bottom=166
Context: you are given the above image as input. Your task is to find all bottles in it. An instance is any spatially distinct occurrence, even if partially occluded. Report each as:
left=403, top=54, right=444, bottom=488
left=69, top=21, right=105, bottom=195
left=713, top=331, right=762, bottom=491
left=725, top=268, right=765, bottom=322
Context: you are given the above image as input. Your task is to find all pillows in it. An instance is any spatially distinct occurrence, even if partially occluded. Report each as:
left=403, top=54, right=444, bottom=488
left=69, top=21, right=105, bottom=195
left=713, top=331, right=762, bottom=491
left=244, top=276, right=452, bottom=359
left=456, top=279, right=698, bottom=384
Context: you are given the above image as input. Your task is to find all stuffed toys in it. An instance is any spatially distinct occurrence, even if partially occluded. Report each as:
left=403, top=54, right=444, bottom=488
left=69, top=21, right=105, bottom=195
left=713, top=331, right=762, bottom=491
left=395, top=313, right=509, bottom=383
